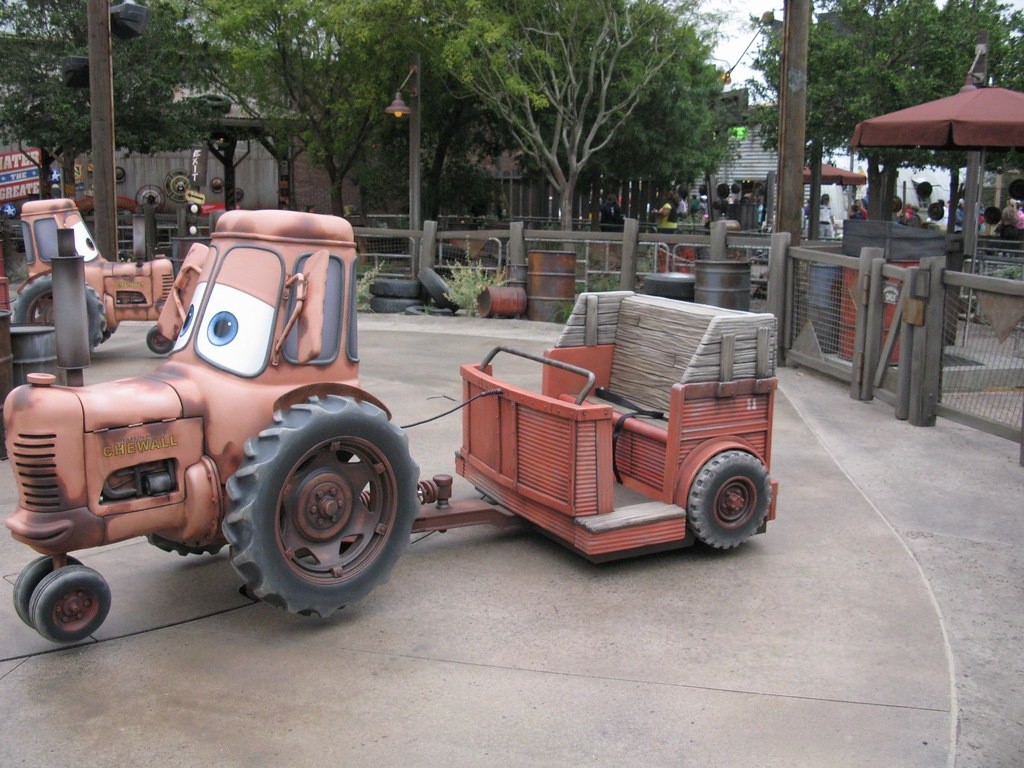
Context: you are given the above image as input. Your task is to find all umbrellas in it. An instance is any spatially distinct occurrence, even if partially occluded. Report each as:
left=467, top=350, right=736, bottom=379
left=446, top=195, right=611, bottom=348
left=775, top=162, right=867, bottom=186
left=850, top=87, right=1024, bottom=347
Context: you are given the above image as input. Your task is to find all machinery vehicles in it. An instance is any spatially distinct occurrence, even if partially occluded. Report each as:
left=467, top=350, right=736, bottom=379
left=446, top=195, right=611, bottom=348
left=3, top=208, right=418, bottom=646
left=12, top=197, right=179, bottom=358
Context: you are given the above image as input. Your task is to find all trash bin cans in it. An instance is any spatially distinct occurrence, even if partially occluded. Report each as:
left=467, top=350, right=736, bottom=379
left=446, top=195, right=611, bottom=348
left=838, top=217, right=946, bottom=367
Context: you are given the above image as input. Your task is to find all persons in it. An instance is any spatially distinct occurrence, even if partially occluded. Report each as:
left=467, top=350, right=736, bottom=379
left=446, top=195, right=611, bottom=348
left=579, top=186, right=752, bottom=229
left=955, top=200, right=985, bottom=234
left=805, top=194, right=834, bottom=236
left=849, top=188, right=869, bottom=220
left=280, top=175, right=290, bottom=210
left=994, top=198, right=1024, bottom=241
left=931, top=200, right=950, bottom=225
left=898, top=204, right=921, bottom=226
left=755, top=196, right=776, bottom=228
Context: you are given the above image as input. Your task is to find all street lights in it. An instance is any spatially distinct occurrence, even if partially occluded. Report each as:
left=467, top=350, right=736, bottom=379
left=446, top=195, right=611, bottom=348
left=383, top=66, right=424, bottom=274
left=958, top=69, right=988, bottom=260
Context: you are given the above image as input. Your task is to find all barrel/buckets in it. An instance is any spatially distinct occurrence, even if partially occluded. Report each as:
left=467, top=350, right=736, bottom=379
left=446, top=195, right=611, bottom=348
left=808, top=263, right=844, bottom=352
left=841, top=258, right=920, bottom=365
left=0, top=309, right=14, bottom=461
left=170, top=234, right=214, bottom=283
left=505, top=263, right=528, bottom=290
left=527, top=249, right=576, bottom=320
left=9, top=326, right=66, bottom=387
left=694, top=260, right=752, bottom=312
left=480, top=286, right=528, bottom=317
